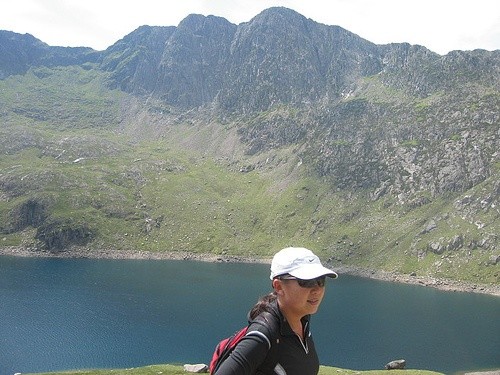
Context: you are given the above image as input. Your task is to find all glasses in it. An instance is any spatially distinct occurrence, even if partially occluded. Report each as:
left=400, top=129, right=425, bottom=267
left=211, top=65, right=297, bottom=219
left=277, top=276, right=324, bottom=288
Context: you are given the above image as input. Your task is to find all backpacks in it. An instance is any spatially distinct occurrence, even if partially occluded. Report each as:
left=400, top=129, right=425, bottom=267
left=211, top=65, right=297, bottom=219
left=209, top=311, right=282, bottom=375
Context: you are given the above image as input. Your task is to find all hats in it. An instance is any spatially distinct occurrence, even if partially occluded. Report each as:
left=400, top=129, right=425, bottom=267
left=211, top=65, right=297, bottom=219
left=269, top=247, right=339, bottom=280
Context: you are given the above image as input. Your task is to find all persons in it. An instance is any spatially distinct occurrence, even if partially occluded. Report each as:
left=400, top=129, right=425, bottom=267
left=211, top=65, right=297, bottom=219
left=208, top=247, right=340, bottom=375
